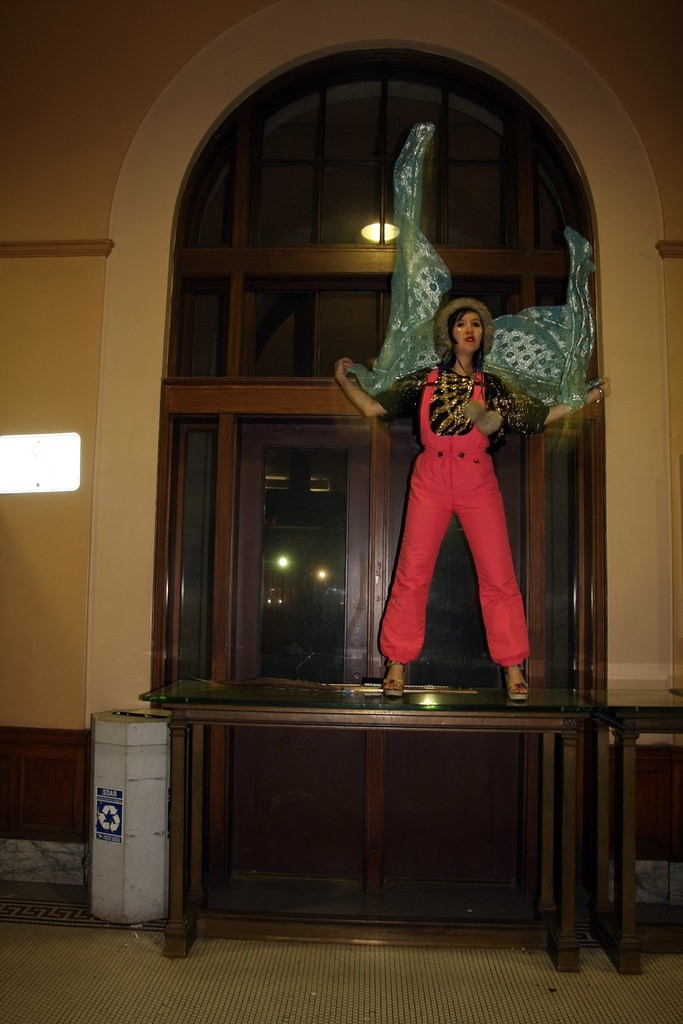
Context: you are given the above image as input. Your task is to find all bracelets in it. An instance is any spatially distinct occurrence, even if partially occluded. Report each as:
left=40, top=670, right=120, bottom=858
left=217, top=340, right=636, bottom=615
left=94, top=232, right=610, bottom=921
left=597, top=386, right=604, bottom=397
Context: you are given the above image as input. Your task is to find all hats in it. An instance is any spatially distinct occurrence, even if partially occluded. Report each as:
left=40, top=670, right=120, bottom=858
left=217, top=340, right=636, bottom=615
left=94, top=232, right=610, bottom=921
left=433, top=298, right=494, bottom=362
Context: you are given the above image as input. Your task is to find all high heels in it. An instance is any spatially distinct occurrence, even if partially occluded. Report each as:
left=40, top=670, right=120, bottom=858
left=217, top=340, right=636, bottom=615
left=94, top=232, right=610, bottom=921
left=383, top=660, right=405, bottom=696
left=503, top=662, right=528, bottom=699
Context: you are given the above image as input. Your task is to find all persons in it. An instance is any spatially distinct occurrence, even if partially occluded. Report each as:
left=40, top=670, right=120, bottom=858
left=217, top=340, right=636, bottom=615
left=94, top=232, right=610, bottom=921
left=334, top=299, right=611, bottom=701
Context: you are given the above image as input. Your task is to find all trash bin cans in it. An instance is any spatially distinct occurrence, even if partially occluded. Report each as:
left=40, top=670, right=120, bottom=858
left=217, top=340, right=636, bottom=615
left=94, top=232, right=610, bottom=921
left=87, top=708, right=174, bottom=924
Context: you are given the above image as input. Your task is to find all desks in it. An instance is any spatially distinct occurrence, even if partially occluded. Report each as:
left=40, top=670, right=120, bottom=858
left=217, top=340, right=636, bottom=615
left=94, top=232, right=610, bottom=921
left=140, top=679, right=683, bottom=977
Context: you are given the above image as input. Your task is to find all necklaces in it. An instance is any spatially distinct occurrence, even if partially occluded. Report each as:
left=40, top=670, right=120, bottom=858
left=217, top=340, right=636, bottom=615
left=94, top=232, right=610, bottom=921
left=457, top=359, right=468, bottom=374
